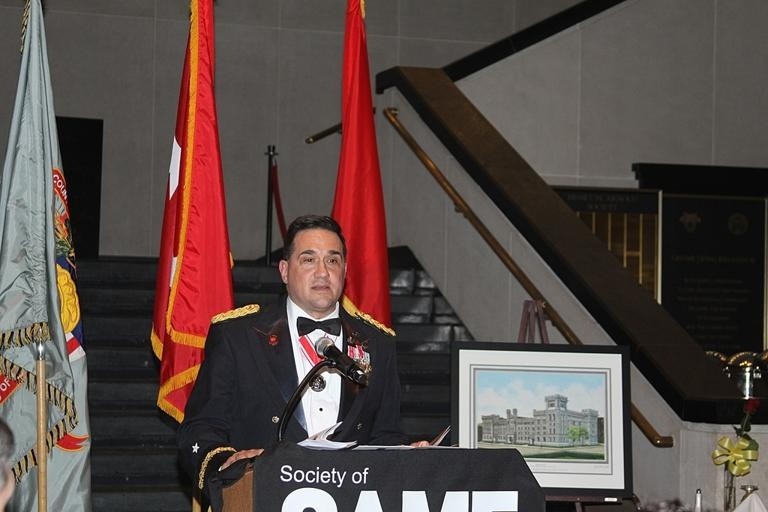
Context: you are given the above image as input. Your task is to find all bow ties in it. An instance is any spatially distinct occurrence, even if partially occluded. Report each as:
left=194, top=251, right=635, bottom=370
left=297, top=317, right=343, bottom=337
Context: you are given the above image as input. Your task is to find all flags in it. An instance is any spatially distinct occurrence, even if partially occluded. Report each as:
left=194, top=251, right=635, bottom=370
left=331, top=0, right=397, bottom=336
left=0, top=0, right=92, bottom=512
left=150, top=0, right=235, bottom=426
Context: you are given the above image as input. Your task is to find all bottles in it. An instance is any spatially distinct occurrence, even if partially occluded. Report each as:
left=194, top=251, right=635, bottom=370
left=693, top=489, right=703, bottom=512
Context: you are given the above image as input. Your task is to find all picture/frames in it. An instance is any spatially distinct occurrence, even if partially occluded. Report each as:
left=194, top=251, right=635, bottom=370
left=449, top=339, right=635, bottom=499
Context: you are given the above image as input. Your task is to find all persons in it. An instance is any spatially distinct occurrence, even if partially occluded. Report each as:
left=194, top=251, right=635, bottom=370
left=176, top=215, right=431, bottom=512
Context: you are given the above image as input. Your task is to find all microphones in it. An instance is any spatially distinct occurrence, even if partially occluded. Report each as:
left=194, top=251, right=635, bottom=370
left=316, top=337, right=366, bottom=385
